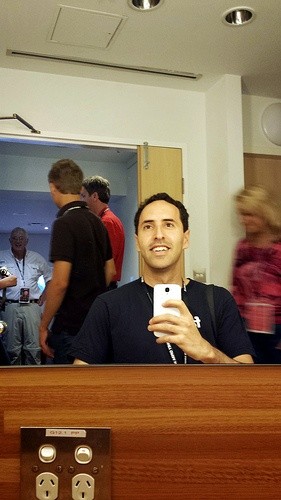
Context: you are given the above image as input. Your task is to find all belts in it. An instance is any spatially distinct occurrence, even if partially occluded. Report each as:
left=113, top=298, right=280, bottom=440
left=4, top=299, right=40, bottom=304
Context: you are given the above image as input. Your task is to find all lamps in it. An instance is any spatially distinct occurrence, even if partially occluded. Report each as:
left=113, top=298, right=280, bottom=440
left=262, top=103, right=281, bottom=146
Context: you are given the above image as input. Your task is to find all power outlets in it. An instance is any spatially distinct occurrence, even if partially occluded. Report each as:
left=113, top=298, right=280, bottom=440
left=19, top=425, right=113, bottom=500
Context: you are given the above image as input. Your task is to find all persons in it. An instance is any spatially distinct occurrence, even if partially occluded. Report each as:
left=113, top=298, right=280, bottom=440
left=20, top=288, right=29, bottom=301
left=232, top=186, right=281, bottom=364
left=69, top=193, right=254, bottom=364
left=0, top=158, right=124, bottom=365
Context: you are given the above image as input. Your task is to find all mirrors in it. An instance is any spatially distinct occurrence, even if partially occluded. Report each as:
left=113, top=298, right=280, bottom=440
left=0, top=0, right=281, bottom=369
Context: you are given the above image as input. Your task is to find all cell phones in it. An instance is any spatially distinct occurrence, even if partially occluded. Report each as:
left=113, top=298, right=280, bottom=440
left=153, top=284, right=181, bottom=338
